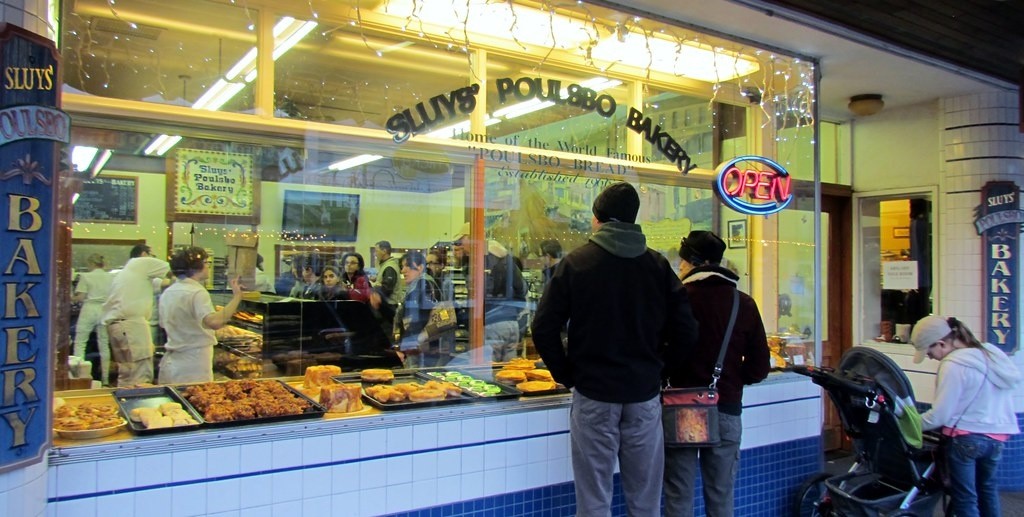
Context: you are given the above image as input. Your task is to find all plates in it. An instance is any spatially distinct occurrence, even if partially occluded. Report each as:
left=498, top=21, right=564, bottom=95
left=53, top=420, right=128, bottom=439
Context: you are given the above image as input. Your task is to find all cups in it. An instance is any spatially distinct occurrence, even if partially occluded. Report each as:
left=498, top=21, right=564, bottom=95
left=896, top=324, right=911, bottom=343
left=81, top=362, right=91, bottom=378
left=881, top=322, right=892, bottom=342
left=770, top=358, right=776, bottom=368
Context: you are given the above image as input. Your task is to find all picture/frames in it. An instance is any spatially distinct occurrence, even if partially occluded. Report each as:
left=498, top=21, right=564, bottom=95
left=727, top=219, right=747, bottom=250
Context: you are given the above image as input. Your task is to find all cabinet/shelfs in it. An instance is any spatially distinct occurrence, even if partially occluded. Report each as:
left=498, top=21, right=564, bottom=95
left=208, top=289, right=404, bottom=380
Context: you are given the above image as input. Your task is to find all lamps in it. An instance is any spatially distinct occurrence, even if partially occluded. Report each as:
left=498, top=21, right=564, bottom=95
left=141, top=37, right=247, bottom=159
left=558, top=76, right=622, bottom=100
left=327, top=154, right=383, bottom=172
left=491, top=96, right=556, bottom=120
left=221, top=15, right=317, bottom=85
left=426, top=114, right=501, bottom=138
left=848, top=94, right=885, bottom=116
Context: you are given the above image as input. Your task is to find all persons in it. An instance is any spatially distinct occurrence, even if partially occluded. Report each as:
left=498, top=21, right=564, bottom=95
left=533, top=183, right=690, bottom=517
left=70, top=223, right=569, bottom=393
left=660, top=228, right=772, bottom=517
left=910, top=316, right=1021, bottom=517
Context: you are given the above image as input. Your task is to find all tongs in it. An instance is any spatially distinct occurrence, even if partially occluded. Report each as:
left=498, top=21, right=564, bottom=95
left=236, top=306, right=255, bottom=318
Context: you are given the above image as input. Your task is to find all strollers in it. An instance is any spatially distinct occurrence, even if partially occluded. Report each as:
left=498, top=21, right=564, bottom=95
left=793, top=345, right=951, bottom=517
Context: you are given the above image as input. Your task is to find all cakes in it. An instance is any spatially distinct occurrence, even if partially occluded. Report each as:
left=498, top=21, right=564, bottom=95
left=302, top=364, right=364, bottom=413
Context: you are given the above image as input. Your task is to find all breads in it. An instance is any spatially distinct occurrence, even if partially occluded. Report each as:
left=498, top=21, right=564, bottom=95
left=767, top=338, right=787, bottom=368
left=130, top=401, right=199, bottom=430
left=51, top=403, right=127, bottom=432
left=359, top=368, right=556, bottom=405
left=213, top=304, right=263, bottom=378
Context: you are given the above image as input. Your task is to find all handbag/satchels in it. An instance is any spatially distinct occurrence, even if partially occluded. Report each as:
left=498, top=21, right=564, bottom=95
left=401, top=279, right=458, bottom=346
left=660, top=385, right=722, bottom=448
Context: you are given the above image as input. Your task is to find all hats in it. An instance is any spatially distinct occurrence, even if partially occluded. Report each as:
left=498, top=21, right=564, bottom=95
left=910, top=314, right=952, bottom=364
left=592, top=181, right=641, bottom=224
left=679, top=230, right=727, bottom=268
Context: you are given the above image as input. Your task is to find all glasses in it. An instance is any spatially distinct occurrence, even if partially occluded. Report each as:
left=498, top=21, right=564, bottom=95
left=426, top=263, right=442, bottom=268
left=202, top=260, right=213, bottom=268
